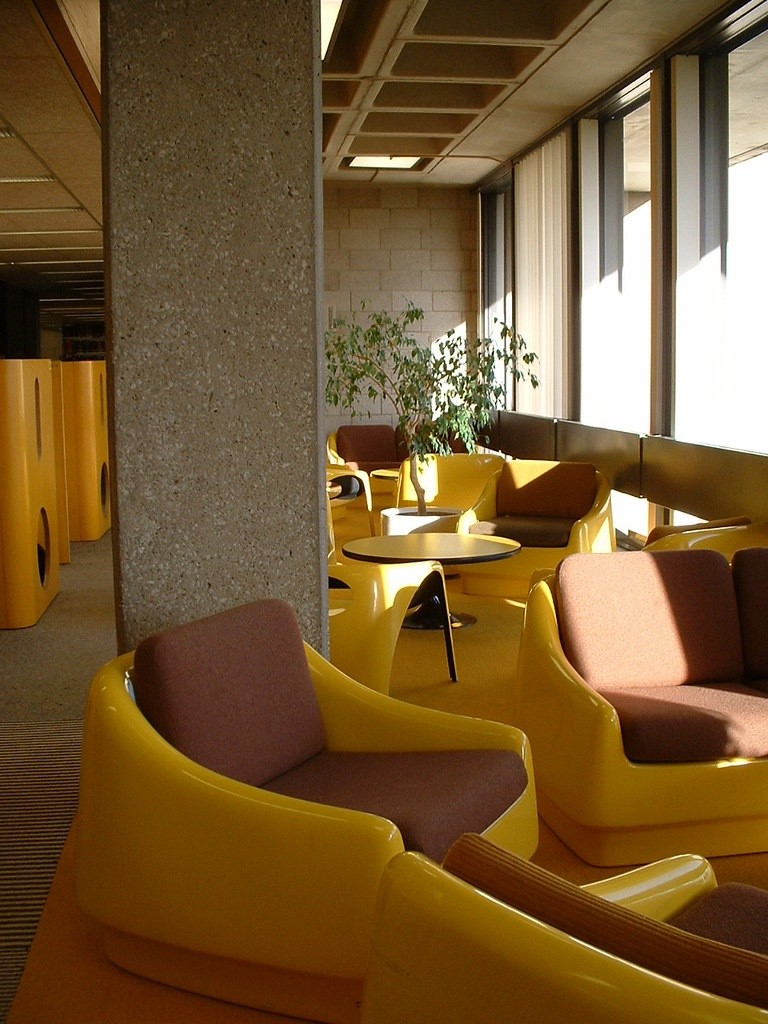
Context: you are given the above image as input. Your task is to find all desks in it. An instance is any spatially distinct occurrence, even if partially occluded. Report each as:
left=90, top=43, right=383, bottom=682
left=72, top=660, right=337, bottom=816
left=372, top=469, right=399, bottom=488
left=344, top=533, right=521, bottom=629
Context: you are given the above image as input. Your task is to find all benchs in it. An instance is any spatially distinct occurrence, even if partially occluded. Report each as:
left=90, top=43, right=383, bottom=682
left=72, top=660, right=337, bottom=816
left=326, top=424, right=468, bottom=496
left=512, top=551, right=767, bottom=865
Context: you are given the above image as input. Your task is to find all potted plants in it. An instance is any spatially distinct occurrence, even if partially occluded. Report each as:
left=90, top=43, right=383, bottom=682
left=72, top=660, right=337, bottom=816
left=324, top=294, right=542, bottom=535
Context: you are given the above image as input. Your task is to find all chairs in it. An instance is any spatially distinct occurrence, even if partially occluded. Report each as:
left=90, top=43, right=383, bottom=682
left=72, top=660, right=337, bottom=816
left=13, top=453, right=768, bottom=1024
